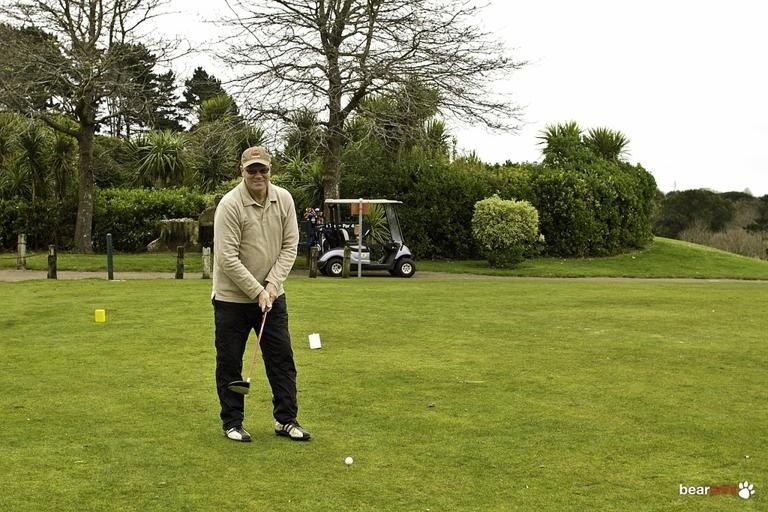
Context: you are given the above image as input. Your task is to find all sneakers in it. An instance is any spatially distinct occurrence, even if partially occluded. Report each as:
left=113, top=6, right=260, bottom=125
left=275, top=419, right=310, bottom=441
left=226, top=426, right=251, bottom=442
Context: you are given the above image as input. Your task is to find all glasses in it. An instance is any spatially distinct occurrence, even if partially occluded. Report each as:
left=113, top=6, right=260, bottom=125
left=245, top=168, right=270, bottom=174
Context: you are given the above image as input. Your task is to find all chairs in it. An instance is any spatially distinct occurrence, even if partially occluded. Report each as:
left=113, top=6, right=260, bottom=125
left=336, top=228, right=371, bottom=252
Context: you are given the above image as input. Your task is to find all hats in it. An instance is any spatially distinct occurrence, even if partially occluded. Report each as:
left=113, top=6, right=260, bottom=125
left=241, top=146, right=270, bottom=169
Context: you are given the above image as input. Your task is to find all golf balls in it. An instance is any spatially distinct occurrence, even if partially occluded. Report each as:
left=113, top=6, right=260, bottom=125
left=345, top=457, right=353, bottom=465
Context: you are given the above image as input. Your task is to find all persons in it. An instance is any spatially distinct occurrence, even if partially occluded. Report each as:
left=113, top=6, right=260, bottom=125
left=210, top=147, right=310, bottom=442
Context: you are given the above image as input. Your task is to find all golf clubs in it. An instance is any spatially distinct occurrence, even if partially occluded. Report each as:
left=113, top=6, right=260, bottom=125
left=223, top=308, right=268, bottom=395
left=305, top=208, right=325, bottom=226
